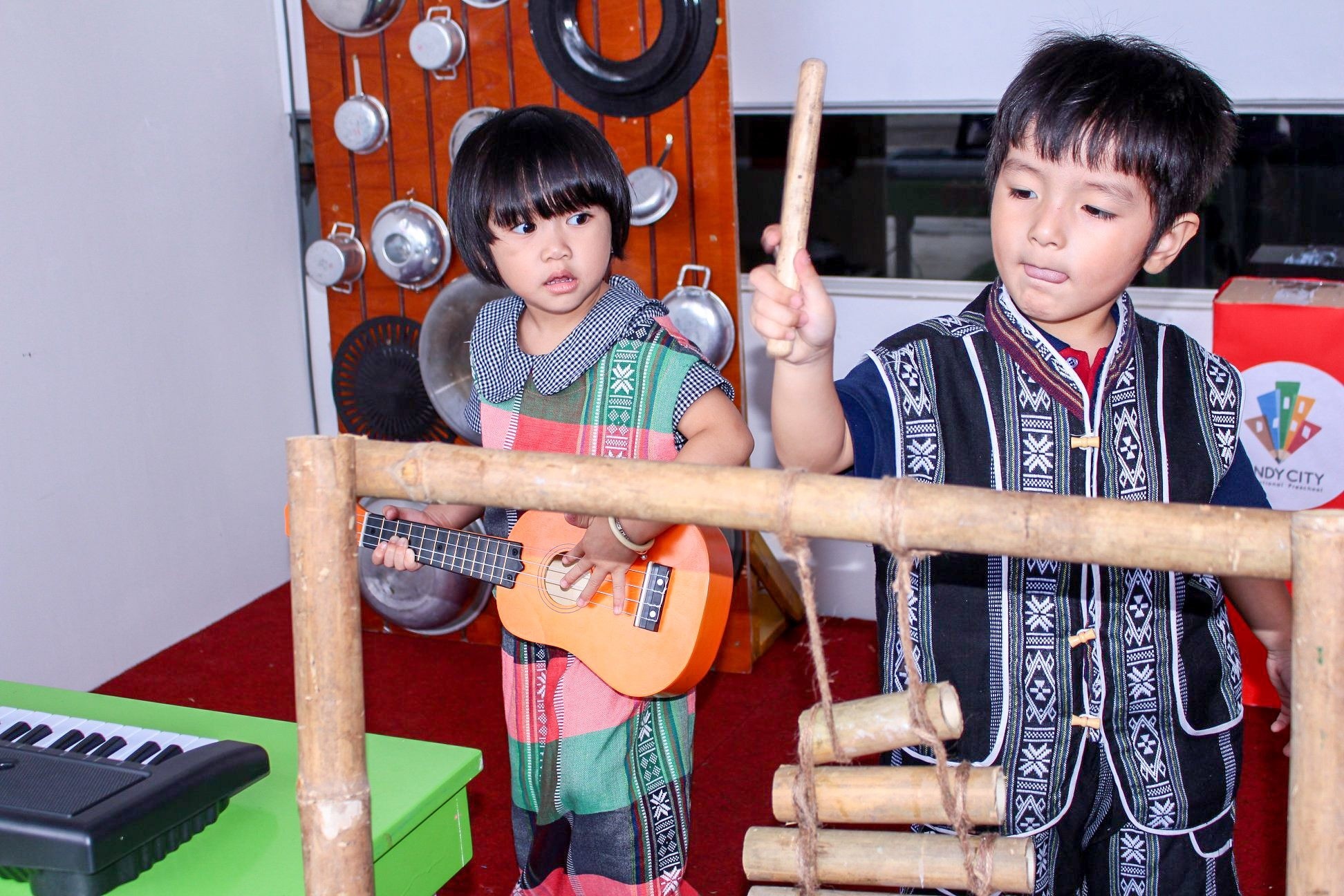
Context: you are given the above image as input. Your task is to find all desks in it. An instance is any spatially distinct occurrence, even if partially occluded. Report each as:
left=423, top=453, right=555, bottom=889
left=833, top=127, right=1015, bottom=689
left=1248, top=244, right=1344, bottom=280
left=0, top=679, right=483, bottom=896
left=847, top=176, right=993, bottom=279
left=1210, top=277, right=1344, bottom=707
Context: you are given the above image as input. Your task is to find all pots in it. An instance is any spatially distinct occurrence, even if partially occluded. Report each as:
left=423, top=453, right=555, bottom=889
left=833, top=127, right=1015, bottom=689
left=294, top=0, right=738, bottom=635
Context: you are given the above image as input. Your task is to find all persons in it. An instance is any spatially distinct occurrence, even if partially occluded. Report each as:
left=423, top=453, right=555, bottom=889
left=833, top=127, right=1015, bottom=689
left=747, top=33, right=1291, bottom=896
left=373, top=107, right=756, bottom=896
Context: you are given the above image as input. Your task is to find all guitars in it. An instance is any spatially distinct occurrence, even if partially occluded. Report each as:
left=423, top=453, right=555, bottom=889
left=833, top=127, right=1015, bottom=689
left=284, top=500, right=733, bottom=700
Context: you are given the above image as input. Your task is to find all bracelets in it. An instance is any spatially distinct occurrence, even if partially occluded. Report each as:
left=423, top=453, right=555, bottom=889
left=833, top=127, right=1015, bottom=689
left=609, top=516, right=655, bottom=556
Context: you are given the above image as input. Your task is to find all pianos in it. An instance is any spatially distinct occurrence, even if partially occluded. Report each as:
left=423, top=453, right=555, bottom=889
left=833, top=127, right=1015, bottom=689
left=0, top=705, right=270, bottom=896
